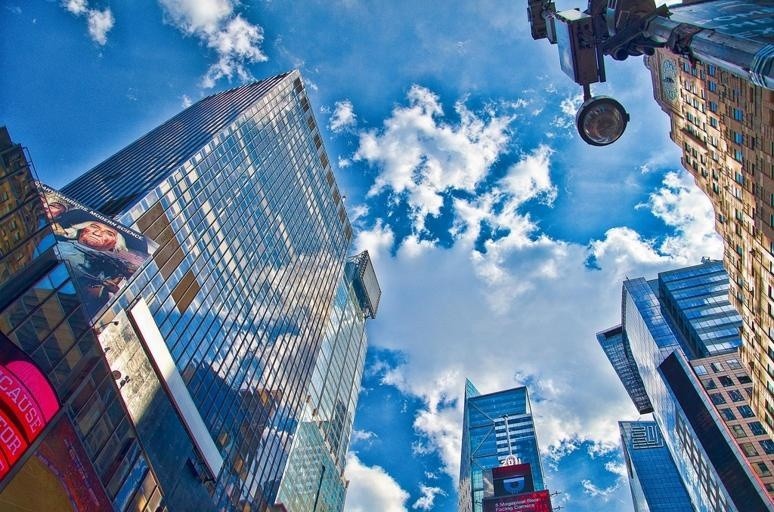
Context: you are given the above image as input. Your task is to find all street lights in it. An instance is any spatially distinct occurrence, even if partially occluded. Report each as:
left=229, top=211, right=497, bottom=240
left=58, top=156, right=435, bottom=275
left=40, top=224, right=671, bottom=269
left=554, top=0, right=773, bottom=145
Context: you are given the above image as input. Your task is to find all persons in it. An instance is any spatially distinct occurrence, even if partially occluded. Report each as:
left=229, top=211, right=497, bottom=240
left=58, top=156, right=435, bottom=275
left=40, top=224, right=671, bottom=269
left=53, top=219, right=132, bottom=297
left=31, top=189, right=75, bottom=236
